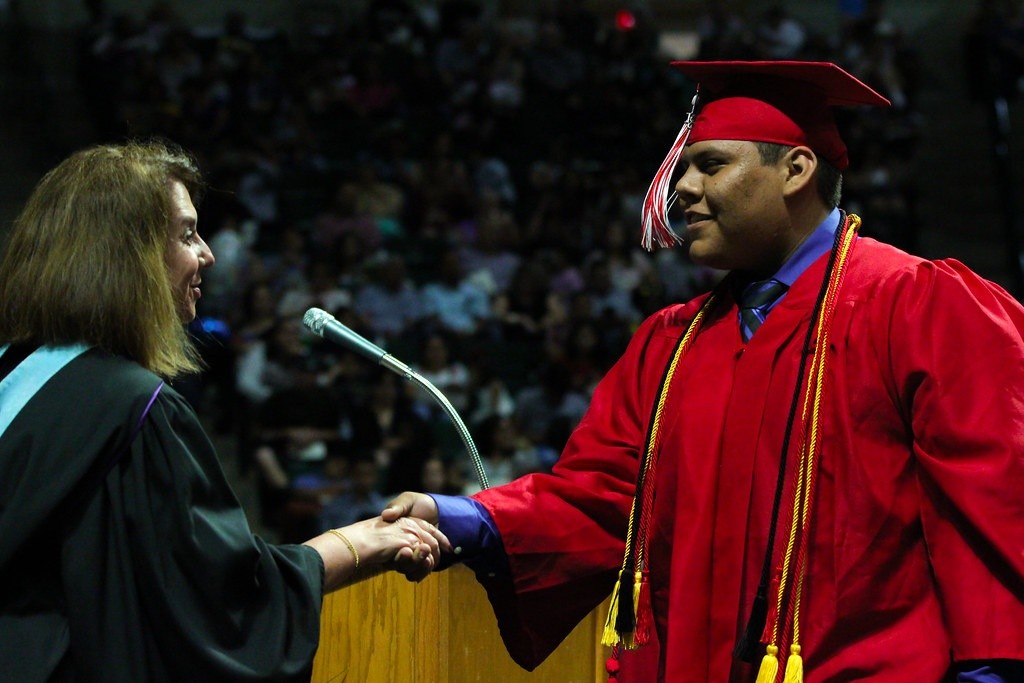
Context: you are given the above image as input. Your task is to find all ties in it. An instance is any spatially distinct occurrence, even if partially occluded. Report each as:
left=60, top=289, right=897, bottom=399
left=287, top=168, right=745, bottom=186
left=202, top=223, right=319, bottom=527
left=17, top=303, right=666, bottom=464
left=736, top=279, right=791, bottom=343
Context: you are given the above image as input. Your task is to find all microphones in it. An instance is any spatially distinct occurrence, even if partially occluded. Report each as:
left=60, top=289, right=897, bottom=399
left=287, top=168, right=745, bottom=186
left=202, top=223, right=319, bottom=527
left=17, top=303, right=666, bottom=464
left=302, top=306, right=491, bottom=490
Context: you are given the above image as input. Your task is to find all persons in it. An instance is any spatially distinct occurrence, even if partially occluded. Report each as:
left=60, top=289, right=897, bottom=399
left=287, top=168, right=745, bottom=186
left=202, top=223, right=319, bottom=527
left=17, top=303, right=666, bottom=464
left=1, top=145, right=455, bottom=683
left=381, top=60, right=1024, bottom=681
left=0, top=1, right=1023, bottom=545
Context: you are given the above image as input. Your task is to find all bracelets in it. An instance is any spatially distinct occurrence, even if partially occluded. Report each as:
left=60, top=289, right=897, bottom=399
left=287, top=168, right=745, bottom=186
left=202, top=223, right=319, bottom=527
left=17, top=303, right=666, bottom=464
left=329, top=529, right=359, bottom=583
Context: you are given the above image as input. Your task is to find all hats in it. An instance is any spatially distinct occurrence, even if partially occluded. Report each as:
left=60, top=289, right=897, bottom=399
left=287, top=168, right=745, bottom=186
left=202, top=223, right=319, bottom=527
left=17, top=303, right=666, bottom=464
left=639, top=61, right=892, bottom=251
